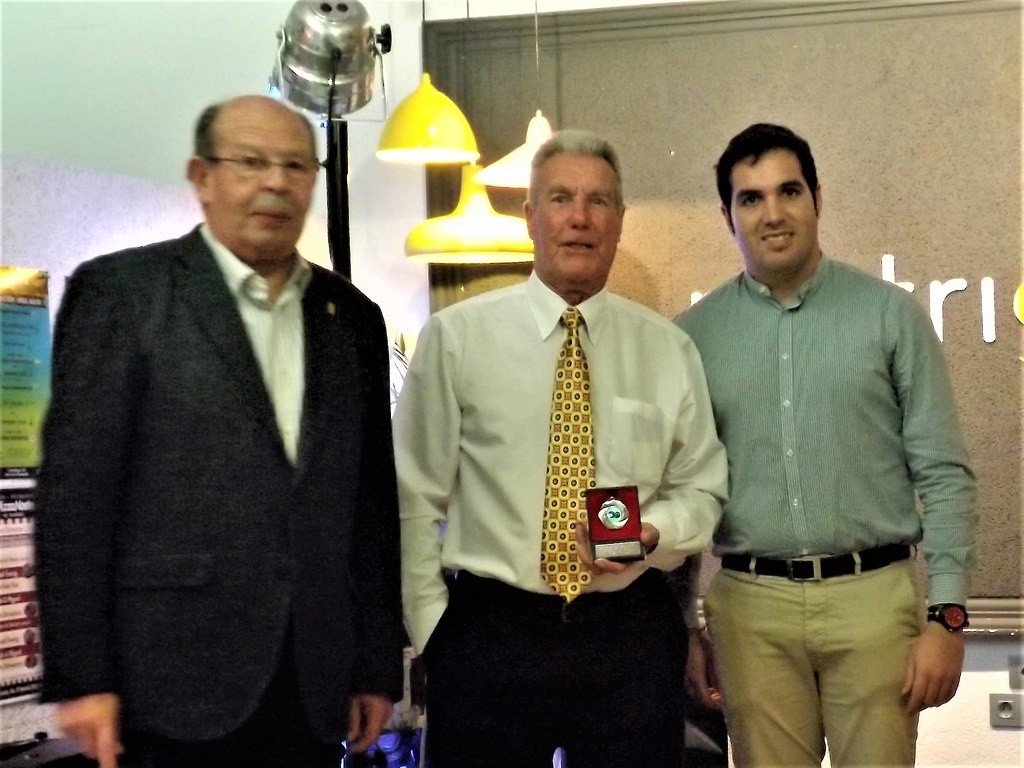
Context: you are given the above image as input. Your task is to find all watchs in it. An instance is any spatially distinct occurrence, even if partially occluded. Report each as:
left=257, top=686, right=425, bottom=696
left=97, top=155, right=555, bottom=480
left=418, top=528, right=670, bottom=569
left=926, top=603, right=969, bottom=632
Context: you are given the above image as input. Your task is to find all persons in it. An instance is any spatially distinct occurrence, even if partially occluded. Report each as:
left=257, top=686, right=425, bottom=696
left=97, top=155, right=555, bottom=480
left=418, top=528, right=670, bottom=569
left=393, top=134, right=728, bottom=768
left=32, top=95, right=406, bottom=768
left=668, top=124, right=975, bottom=768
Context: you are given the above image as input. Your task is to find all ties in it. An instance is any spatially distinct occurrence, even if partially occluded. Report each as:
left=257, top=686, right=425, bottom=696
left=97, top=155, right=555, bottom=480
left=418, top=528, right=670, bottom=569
left=539, top=306, right=596, bottom=603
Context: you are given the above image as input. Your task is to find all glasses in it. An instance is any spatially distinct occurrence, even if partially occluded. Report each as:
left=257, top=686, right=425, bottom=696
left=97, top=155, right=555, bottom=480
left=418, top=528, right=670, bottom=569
left=202, top=155, right=318, bottom=179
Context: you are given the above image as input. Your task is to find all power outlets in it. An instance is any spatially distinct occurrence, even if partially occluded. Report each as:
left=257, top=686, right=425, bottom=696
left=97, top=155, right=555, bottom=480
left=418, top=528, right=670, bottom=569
left=989, top=693, right=1024, bottom=727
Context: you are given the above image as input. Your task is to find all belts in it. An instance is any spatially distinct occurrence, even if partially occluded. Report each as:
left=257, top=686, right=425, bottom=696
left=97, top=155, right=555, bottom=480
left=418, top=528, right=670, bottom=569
left=457, top=568, right=688, bottom=623
left=722, top=542, right=911, bottom=582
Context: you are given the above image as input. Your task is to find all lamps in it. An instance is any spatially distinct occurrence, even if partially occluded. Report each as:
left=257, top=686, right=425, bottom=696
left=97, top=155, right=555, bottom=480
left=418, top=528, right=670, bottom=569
left=404, top=0, right=536, bottom=265
left=473, top=0, right=552, bottom=188
left=374, top=1, right=482, bottom=166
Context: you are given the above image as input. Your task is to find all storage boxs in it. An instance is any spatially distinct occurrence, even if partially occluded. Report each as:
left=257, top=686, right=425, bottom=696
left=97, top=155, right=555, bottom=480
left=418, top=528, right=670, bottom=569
left=586, top=485, right=645, bottom=564
